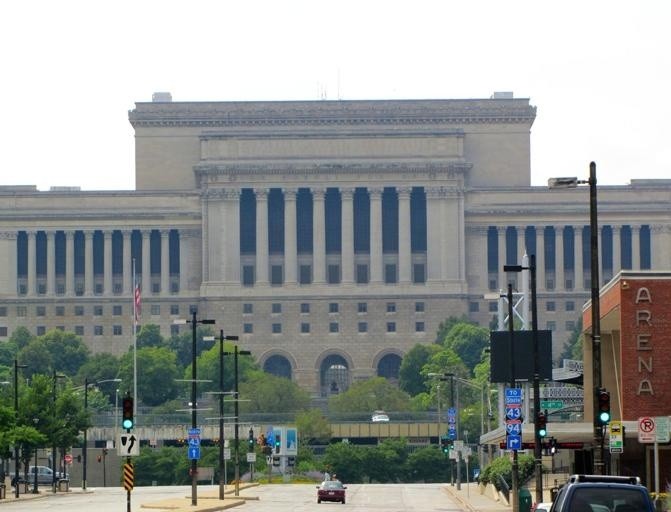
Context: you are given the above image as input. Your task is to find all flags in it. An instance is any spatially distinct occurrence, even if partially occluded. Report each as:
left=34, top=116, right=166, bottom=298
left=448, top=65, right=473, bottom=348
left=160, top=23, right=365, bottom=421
left=134, top=272, right=143, bottom=324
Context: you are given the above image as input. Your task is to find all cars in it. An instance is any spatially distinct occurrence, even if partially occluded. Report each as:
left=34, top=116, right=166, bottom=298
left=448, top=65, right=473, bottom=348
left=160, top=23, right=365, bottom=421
left=369, top=414, right=389, bottom=424
left=549, top=474, right=656, bottom=512
left=315, top=480, right=348, bottom=504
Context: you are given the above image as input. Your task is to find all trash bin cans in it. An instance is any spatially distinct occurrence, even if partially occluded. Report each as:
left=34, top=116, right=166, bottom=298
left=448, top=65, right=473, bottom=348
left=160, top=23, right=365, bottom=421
left=18, top=479, right=29, bottom=494
left=58, top=479, right=69, bottom=492
left=152, top=479, right=157, bottom=486
left=519, top=488, right=532, bottom=512
left=0, top=482, right=6, bottom=499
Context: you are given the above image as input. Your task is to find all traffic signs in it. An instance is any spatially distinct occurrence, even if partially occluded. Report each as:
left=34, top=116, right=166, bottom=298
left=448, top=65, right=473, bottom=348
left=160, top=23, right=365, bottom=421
left=188, top=435, right=201, bottom=446
left=507, top=423, right=522, bottom=435
left=506, top=406, right=521, bottom=419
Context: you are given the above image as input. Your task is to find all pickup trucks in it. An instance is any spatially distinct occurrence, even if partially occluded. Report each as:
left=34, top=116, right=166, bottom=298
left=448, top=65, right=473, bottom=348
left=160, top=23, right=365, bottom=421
left=9, top=466, right=71, bottom=488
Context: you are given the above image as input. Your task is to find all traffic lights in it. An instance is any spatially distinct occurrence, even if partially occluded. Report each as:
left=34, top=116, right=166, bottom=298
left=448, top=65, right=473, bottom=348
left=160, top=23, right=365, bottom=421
left=275, top=435, right=280, bottom=454
left=122, top=397, right=133, bottom=429
left=538, top=416, right=546, bottom=437
left=249, top=439, right=254, bottom=452
left=598, top=391, right=611, bottom=422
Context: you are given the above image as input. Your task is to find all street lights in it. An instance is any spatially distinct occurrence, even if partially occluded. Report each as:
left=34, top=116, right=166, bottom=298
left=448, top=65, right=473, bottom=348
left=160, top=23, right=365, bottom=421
left=548, top=162, right=604, bottom=477
left=186, top=303, right=216, bottom=506
left=82, top=377, right=123, bottom=490
left=215, top=329, right=250, bottom=499
left=426, top=373, right=494, bottom=472
left=14, top=358, right=28, bottom=500
left=500, top=285, right=522, bottom=512
left=52, top=366, right=69, bottom=494
left=503, top=254, right=542, bottom=501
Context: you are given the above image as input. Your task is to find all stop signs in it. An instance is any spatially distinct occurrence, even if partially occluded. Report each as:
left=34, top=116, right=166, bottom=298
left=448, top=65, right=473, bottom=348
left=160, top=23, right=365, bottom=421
left=65, top=455, right=72, bottom=464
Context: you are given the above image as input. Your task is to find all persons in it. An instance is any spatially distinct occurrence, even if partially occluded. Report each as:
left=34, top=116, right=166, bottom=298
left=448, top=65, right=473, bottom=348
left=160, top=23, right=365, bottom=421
left=663, top=475, right=671, bottom=492
left=324, top=470, right=338, bottom=481
